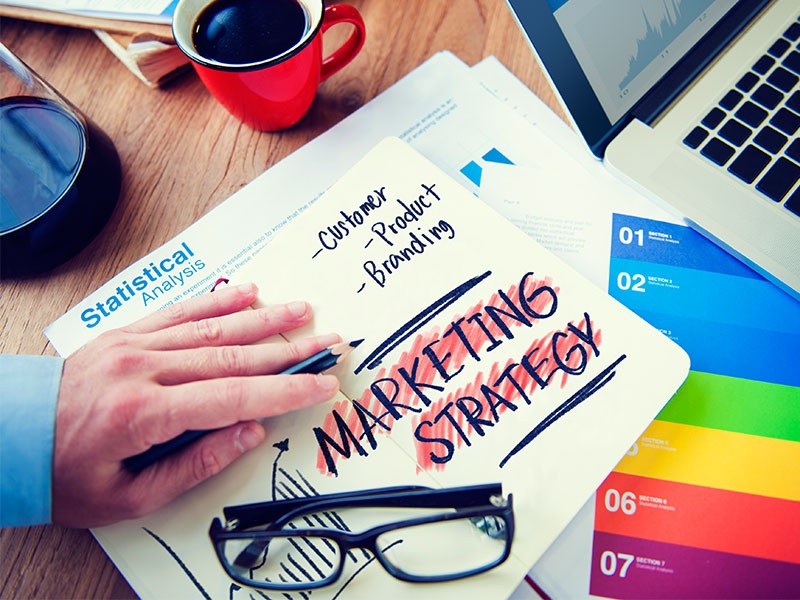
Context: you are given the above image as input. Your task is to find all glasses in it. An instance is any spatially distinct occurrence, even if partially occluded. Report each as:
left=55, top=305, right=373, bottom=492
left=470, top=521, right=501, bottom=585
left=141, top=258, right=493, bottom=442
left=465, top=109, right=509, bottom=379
left=208, top=483, right=515, bottom=590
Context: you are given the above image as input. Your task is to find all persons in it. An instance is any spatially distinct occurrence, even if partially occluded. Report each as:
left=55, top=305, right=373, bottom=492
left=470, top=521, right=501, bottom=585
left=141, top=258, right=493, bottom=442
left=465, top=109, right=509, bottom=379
left=1, top=284, right=343, bottom=530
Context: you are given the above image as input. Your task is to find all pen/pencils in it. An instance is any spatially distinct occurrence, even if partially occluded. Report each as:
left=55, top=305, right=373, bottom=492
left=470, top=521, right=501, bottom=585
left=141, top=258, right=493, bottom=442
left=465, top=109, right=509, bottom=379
left=121, top=338, right=365, bottom=474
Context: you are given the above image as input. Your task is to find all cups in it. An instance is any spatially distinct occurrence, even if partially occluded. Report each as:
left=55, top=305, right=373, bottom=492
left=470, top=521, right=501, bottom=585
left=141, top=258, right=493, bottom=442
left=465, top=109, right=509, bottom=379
left=1, top=42, right=125, bottom=279
left=170, top=0, right=367, bottom=134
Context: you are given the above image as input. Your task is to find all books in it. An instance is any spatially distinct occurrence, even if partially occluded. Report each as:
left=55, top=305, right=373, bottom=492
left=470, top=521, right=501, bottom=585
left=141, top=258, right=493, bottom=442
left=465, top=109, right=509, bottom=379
left=94, top=27, right=189, bottom=86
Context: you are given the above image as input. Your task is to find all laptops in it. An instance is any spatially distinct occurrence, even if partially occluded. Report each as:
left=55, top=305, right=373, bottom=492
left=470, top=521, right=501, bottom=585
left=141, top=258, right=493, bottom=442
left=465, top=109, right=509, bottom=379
left=507, top=1, right=800, bottom=306
